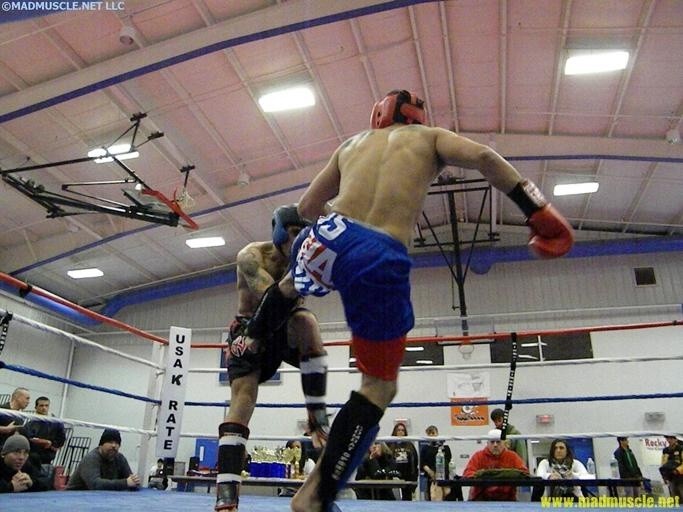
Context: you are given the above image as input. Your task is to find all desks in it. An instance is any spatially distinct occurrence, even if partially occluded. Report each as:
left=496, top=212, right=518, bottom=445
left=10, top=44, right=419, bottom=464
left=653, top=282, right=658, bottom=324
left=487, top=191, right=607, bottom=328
left=433, top=478, right=645, bottom=502
left=166, top=475, right=423, bottom=502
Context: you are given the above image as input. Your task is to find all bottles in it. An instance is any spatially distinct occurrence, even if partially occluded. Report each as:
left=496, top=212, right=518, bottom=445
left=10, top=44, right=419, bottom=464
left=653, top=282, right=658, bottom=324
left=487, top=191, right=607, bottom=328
left=436, top=448, right=444, bottom=479
left=608, top=456, right=621, bottom=480
left=448, top=457, right=456, bottom=479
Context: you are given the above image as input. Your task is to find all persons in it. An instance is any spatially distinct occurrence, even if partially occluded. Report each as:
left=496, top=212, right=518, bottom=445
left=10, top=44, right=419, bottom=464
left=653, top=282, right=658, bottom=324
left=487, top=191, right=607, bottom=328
left=660, top=435, right=683, bottom=503
left=613, top=437, right=643, bottom=499
left=534, top=438, right=590, bottom=503
left=464, top=429, right=530, bottom=501
left=0, top=388, right=30, bottom=447
left=66, top=428, right=142, bottom=491
left=491, top=409, right=528, bottom=469
left=283, top=422, right=452, bottom=499
left=148, top=457, right=168, bottom=490
left=26, top=396, right=66, bottom=464
left=213, top=206, right=328, bottom=512
left=0, top=434, right=48, bottom=493
left=229, top=86, right=575, bottom=511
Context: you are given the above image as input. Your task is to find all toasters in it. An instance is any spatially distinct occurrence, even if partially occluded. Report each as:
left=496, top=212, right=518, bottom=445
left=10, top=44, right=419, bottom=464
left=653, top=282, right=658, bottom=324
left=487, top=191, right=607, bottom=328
left=584, top=457, right=595, bottom=479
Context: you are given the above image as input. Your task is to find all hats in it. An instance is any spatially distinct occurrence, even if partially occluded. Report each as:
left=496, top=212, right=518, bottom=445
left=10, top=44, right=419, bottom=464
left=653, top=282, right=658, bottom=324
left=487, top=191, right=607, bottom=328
left=98, top=427, right=122, bottom=444
left=1, top=434, right=30, bottom=453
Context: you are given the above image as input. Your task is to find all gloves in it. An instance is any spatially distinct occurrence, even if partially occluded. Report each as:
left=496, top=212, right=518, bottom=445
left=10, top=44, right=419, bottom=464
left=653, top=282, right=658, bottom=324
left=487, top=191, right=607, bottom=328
left=526, top=203, right=575, bottom=258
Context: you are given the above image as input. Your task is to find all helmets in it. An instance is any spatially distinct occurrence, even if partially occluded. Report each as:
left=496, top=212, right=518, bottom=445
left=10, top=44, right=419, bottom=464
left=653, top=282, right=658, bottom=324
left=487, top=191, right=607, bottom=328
left=369, top=90, right=429, bottom=129
left=272, top=202, right=310, bottom=258
